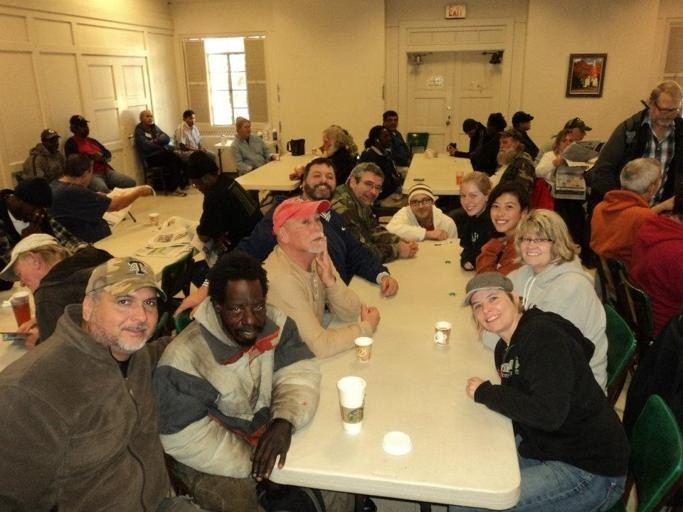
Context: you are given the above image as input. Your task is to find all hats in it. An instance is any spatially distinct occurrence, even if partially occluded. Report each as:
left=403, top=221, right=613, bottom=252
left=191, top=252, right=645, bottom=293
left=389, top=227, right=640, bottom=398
left=70, top=114, right=90, bottom=128
left=463, top=119, right=477, bottom=132
left=13, top=175, right=53, bottom=209
left=564, top=119, right=592, bottom=131
left=0, top=232, right=60, bottom=281
left=84, top=255, right=168, bottom=303
left=271, top=197, right=332, bottom=236
left=512, top=111, right=534, bottom=122
left=41, top=128, right=61, bottom=141
left=460, top=272, right=514, bottom=307
left=407, top=183, right=435, bottom=206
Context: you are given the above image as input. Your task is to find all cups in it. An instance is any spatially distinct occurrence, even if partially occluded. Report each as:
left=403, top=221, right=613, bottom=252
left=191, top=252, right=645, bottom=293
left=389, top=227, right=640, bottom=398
left=275, top=154, right=280, bottom=161
left=456, top=171, right=464, bottom=185
left=433, top=321, right=451, bottom=347
left=355, top=336, right=373, bottom=364
left=337, top=376, right=367, bottom=436
left=148, top=213, right=160, bottom=225
left=10, top=291, right=31, bottom=328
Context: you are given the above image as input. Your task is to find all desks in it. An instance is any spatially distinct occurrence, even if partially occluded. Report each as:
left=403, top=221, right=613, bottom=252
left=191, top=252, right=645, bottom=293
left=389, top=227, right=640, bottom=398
left=216, top=140, right=278, bottom=176
left=0, top=216, right=213, bottom=374
left=235, top=151, right=324, bottom=191
left=266, top=238, right=521, bottom=511
left=403, top=151, right=476, bottom=195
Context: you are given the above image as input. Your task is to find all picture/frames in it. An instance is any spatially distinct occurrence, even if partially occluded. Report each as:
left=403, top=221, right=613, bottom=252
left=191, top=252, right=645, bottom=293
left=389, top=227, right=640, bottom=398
left=565, top=53, right=608, bottom=99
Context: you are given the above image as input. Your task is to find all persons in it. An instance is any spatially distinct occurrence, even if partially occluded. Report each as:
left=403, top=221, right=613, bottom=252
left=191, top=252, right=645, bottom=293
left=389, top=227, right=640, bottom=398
left=565, top=116, right=592, bottom=140
left=172, top=158, right=399, bottom=320
left=173, top=110, right=215, bottom=163
left=153, top=253, right=322, bottom=512
left=359, top=125, right=407, bottom=199
left=535, top=129, right=596, bottom=268
left=230, top=115, right=273, bottom=177
left=23, top=128, right=66, bottom=183
left=459, top=171, right=506, bottom=270
left=511, top=112, right=539, bottom=161
left=1, top=233, right=115, bottom=348
left=50, top=152, right=154, bottom=250
left=0, top=256, right=204, bottom=512
left=62, top=113, right=136, bottom=193
left=489, top=128, right=524, bottom=187
left=487, top=112, right=506, bottom=143
left=311, top=124, right=359, bottom=159
left=0, top=176, right=86, bottom=290
left=260, top=198, right=381, bottom=361
left=328, top=162, right=419, bottom=262
left=382, top=111, right=413, bottom=168
left=590, top=158, right=675, bottom=274
left=289, top=126, right=357, bottom=186
left=447, top=109, right=494, bottom=173
left=476, top=180, right=529, bottom=276
left=385, top=183, right=457, bottom=243
left=133, top=108, right=187, bottom=197
left=449, top=272, right=631, bottom=512
left=588, top=80, right=683, bottom=213
left=479, top=209, right=609, bottom=399
left=628, top=179, right=683, bottom=341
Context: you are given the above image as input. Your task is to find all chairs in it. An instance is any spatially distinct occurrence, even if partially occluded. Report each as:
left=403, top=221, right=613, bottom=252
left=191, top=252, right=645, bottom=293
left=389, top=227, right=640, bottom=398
left=610, top=395, right=683, bottom=512
left=604, top=302, right=636, bottom=407
left=149, top=312, right=169, bottom=343
left=619, top=272, right=655, bottom=376
left=596, top=249, right=625, bottom=304
left=175, top=307, right=192, bottom=332
left=408, top=132, right=430, bottom=152
left=133, top=142, right=171, bottom=196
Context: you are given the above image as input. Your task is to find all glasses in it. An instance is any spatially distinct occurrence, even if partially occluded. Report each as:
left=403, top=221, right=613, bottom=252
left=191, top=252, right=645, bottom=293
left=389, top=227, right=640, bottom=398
left=358, top=179, right=382, bottom=193
left=654, top=101, right=682, bottom=113
left=409, top=198, right=431, bottom=205
left=518, top=236, right=552, bottom=243
